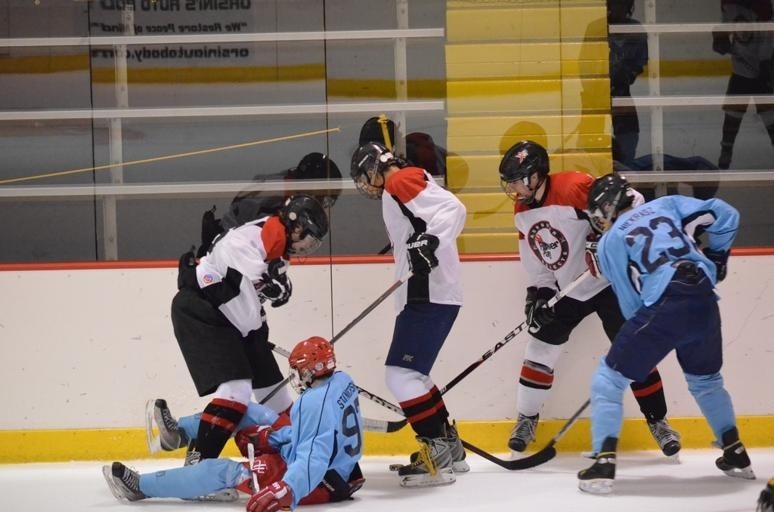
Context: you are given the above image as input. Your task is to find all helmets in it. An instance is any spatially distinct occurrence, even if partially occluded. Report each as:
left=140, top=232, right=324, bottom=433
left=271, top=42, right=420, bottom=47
left=287, top=153, right=341, bottom=208
left=276, top=194, right=328, bottom=253
left=288, top=336, right=336, bottom=382
left=588, top=173, right=636, bottom=224
left=498, top=140, right=550, bottom=183
left=350, top=142, right=408, bottom=182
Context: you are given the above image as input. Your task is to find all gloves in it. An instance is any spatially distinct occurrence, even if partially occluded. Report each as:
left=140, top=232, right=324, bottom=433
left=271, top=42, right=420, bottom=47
left=406, top=232, right=438, bottom=278
left=525, top=286, right=556, bottom=333
left=247, top=481, right=292, bottom=512
left=702, top=247, right=730, bottom=280
left=585, top=234, right=602, bottom=278
left=256, top=273, right=293, bottom=308
left=235, top=425, right=277, bottom=458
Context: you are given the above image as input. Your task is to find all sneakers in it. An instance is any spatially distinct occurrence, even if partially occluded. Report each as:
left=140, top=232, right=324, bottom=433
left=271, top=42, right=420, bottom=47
left=716, top=427, right=750, bottom=470
left=154, top=399, right=187, bottom=450
left=399, top=423, right=465, bottom=474
left=112, top=462, right=151, bottom=502
left=577, top=437, right=616, bottom=479
left=647, top=416, right=680, bottom=456
left=509, top=412, right=539, bottom=451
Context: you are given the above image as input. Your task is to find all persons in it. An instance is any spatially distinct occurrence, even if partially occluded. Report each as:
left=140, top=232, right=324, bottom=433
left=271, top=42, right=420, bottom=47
left=571, top=170, right=753, bottom=484
left=347, top=142, right=471, bottom=478
left=358, top=1, right=774, bottom=193
left=109, top=335, right=364, bottom=512
left=495, top=136, right=682, bottom=457
left=184, top=144, right=342, bottom=262
left=170, top=193, right=330, bottom=461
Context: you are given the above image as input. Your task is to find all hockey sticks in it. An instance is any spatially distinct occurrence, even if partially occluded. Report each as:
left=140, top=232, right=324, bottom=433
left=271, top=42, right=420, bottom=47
left=360, top=269, right=593, bottom=431
left=267, top=341, right=558, bottom=470
left=262, top=271, right=412, bottom=407
left=527, top=399, right=591, bottom=469
